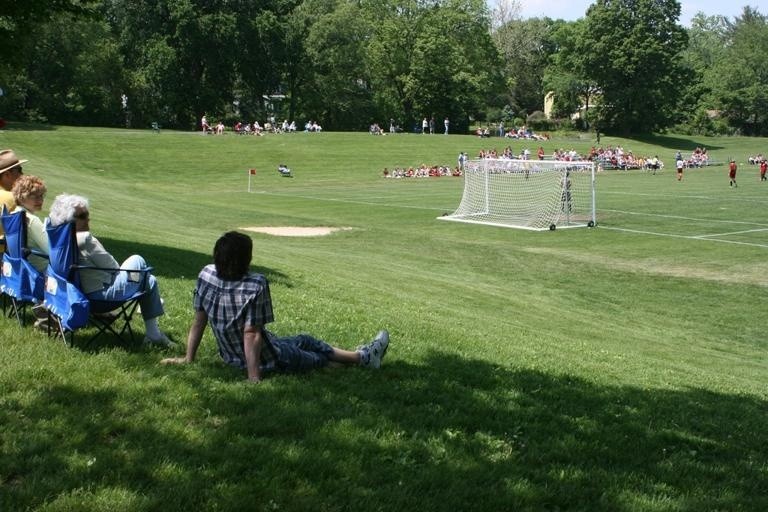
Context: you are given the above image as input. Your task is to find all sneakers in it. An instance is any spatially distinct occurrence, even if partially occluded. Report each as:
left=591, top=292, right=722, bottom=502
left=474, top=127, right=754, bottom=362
left=143, top=334, right=178, bottom=352
left=355, top=328, right=391, bottom=372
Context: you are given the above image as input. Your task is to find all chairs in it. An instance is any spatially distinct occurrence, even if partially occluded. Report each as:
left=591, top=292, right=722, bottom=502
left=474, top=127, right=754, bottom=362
left=0, top=203, right=51, bottom=328
left=34, top=217, right=153, bottom=353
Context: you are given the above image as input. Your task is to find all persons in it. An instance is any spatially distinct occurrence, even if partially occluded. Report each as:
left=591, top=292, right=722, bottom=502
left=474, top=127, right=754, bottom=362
left=245, top=124, right=253, bottom=136
left=475, top=120, right=550, bottom=141
left=159, top=230, right=390, bottom=384
left=675, top=146, right=707, bottom=179
left=215, top=121, right=225, bottom=135
left=202, top=115, right=209, bottom=137
left=44, top=191, right=182, bottom=353
left=371, top=123, right=386, bottom=136
left=383, top=164, right=461, bottom=177
left=312, top=121, right=322, bottom=132
left=457, top=146, right=664, bottom=174
left=6, top=173, right=58, bottom=272
left=305, top=121, right=314, bottom=131
left=0, top=148, right=32, bottom=261
left=235, top=122, right=242, bottom=131
left=759, top=160, right=767, bottom=181
left=558, top=171, right=573, bottom=213
left=443, top=116, right=450, bottom=134
left=288, top=120, right=297, bottom=133
left=421, top=117, right=429, bottom=135
left=281, top=119, right=289, bottom=132
left=264, top=119, right=272, bottom=133
left=749, top=153, right=763, bottom=165
left=727, top=159, right=738, bottom=189
left=389, top=118, right=396, bottom=134
left=253, top=121, right=263, bottom=136
left=272, top=123, right=282, bottom=135
left=428, top=117, right=436, bottom=135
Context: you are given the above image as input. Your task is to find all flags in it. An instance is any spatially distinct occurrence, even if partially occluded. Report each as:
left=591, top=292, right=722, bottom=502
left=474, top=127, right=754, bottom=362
left=249, top=169, right=256, bottom=175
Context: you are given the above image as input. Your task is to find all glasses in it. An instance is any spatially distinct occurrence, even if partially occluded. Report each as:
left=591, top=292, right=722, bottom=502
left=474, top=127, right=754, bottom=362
left=25, top=193, right=45, bottom=200
left=11, top=166, right=22, bottom=172
left=69, top=211, right=89, bottom=220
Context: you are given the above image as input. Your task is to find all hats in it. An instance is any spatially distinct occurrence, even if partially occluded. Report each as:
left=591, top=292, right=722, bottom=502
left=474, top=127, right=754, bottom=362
left=0, top=149, right=30, bottom=175
